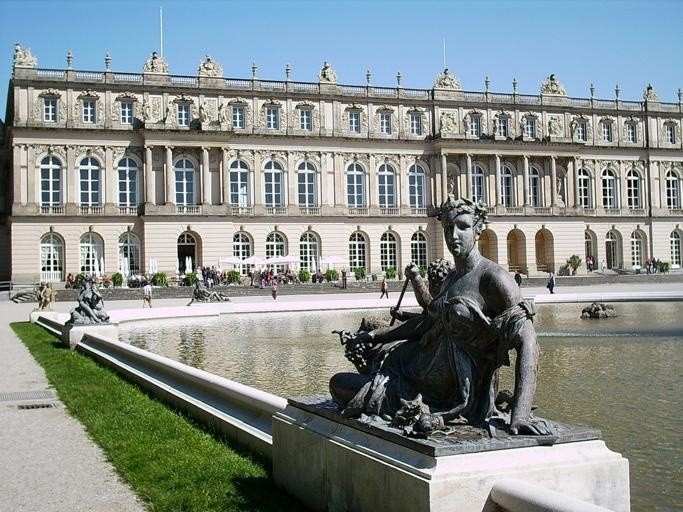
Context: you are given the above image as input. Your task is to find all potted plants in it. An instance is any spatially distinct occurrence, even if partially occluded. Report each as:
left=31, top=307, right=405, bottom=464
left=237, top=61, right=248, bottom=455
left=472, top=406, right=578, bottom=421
left=150, top=272, right=169, bottom=288
left=111, top=272, right=123, bottom=288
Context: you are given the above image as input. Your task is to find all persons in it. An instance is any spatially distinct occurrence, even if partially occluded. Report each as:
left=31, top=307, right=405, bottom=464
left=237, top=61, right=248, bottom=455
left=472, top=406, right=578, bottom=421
left=514, top=269, right=522, bottom=286
left=379, top=278, right=389, bottom=299
left=547, top=74, right=561, bottom=95
left=203, top=55, right=217, bottom=77
left=586, top=255, right=607, bottom=275
left=643, top=257, right=664, bottom=275
left=440, top=69, right=455, bottom=89
left=271, top=282, right=278, bottom=300
left=321, top=61, right=334, bottom=83
left=150, top=51, right=159, bottom=71
left=187, top=281, right=231, bottom=306
left=328, top=255, right=458, bottom=420
left=70, top=287, right=110, bottom=324
left=547, top=271, right=556, bottom=295
left=13, top=43, right=25, bottom=65
left=143, top=281, right=152, bottom=309
left=36, top=282, right=58, bottom=311
left=645, top=83, right=657, bottom=100
left=65, top=271, right=154, bottom=290
left=331, top=192, right=560, bottom=438
left=176, top=265, right=350, bottom=289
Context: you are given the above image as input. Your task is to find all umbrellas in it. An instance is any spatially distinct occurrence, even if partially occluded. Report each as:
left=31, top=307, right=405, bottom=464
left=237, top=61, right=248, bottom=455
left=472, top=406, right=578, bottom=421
left=240, top=255, right=267, bottom=271
left=219, top=255, right=242, bottom=271
left=276, top=253, right=303, bottom=271
left=93, top=256, right=194, bottom=277
left=322, top=254, right=348, bottom=272
left=264, top=255, right=285, bottom=272
left=317, top=257, right=323, bottom=274
left=310, top=255, right=317, bottom=274
